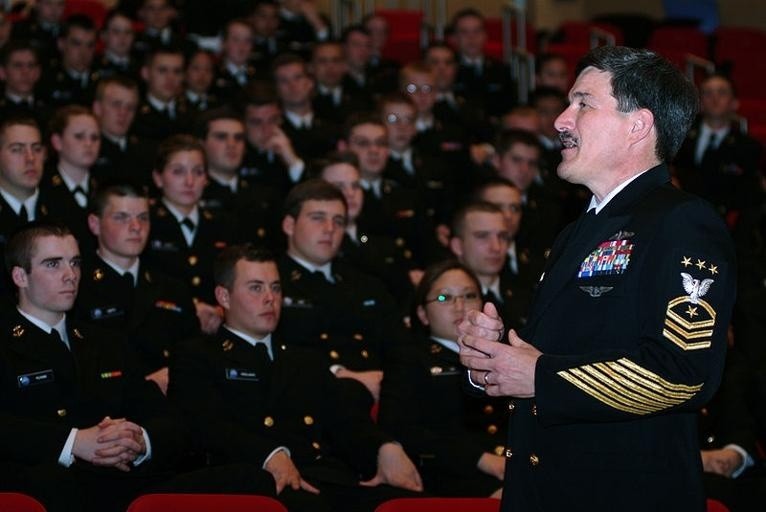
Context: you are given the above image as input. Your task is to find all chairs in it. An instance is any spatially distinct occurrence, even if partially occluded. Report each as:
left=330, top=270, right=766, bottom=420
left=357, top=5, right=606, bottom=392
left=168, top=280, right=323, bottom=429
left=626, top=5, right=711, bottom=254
left=4, top=1, right=163, bottom=40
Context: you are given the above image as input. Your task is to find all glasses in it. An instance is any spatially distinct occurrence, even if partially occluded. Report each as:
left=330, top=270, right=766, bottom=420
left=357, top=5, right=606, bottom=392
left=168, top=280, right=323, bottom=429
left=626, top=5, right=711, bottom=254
left=406, top=83, right=432, bottom=94
left=386, top=113, right=412, bottom=124
left=424, top=292, right=478, bottom=304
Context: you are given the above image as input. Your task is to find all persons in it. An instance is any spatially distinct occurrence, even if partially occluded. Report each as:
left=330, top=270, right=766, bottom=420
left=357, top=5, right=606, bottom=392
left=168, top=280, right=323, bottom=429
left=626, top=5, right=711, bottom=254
left=669, top=69, right=766, bottom=511
left=457, top=46, right=730, bottom=512
left=0, top=0, right=593, bottom=512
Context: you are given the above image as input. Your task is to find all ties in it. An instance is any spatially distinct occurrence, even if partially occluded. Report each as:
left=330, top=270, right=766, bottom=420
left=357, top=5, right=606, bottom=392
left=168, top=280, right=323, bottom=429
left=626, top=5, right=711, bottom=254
left=702, top=132, right=717, bottom=163
left=123, top=271, right=134, bottom=286
left=255, top=341, right=271, bottom=363
left=51, top=327, right=69, bottom=359
